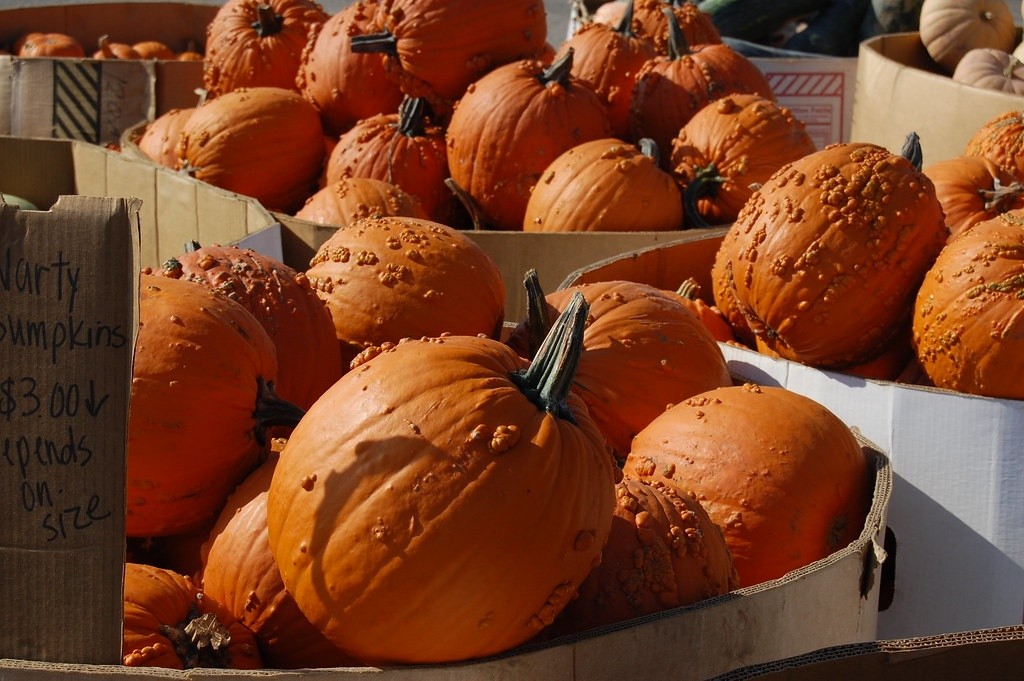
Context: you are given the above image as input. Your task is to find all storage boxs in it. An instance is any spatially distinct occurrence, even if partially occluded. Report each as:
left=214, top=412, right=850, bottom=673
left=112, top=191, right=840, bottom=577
left=0, top=0, right=1024, bottom=681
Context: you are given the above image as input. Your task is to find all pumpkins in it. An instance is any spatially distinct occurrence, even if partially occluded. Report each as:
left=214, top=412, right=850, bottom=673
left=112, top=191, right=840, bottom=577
left=0, top=0, right=1024, bottom=669
